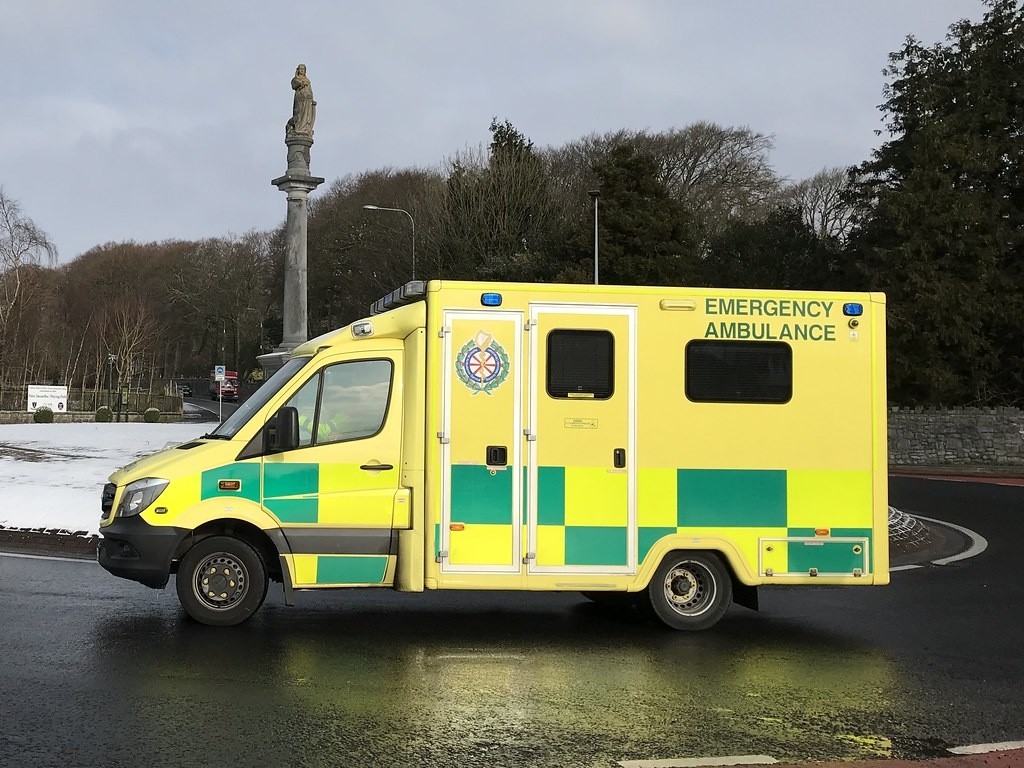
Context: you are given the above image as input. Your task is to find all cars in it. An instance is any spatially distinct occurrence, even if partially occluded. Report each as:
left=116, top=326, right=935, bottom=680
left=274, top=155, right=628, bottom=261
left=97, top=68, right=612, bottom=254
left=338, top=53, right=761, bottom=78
left=181, top=384, right=192, bottom=397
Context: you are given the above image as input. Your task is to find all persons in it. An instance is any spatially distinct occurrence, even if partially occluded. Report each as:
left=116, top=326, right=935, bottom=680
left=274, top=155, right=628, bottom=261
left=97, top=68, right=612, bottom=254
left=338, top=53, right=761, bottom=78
left=294, top=374, right=364, bottom=443
left=291, top=64, right=313, bottom=133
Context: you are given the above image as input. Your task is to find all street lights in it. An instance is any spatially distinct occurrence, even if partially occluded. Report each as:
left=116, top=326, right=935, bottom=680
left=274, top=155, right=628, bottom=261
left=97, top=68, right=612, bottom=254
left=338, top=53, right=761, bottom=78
left=363, top=205, right=416, bottom=282
left=588, top=190, right=601, bottom=285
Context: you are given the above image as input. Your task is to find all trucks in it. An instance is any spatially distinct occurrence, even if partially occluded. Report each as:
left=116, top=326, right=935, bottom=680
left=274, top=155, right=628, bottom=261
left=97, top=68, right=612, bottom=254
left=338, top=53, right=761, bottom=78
left=96, top=275, right=892, bottom=637
left=209, top=369, right=240, bottom=403
